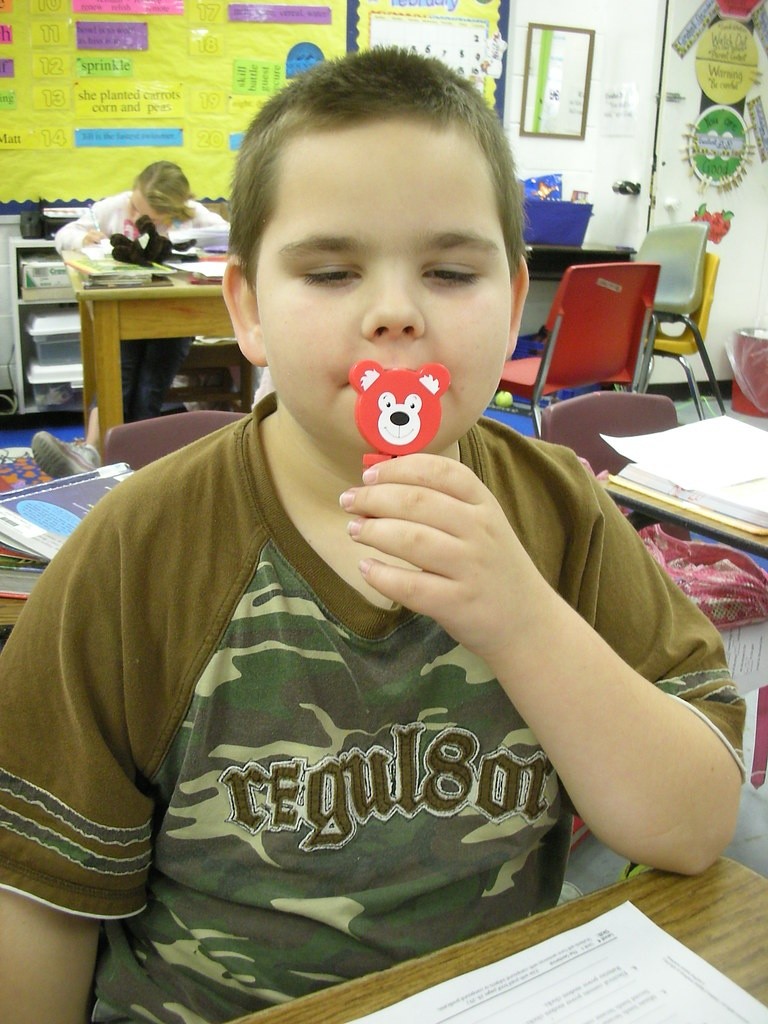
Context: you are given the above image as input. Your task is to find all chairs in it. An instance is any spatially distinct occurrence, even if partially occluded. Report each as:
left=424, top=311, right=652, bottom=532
left=497, top=221, right=722, bottom=541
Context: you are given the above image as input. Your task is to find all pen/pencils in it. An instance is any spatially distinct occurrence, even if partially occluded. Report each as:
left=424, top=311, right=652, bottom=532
left=88, top=204, right=100, bottom=232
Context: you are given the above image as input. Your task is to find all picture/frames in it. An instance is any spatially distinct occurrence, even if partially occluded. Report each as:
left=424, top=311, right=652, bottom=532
left=519, top=22, right=595, bottom=140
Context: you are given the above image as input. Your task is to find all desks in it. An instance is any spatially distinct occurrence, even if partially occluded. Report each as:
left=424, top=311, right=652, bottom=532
left=223, top=857, right=768, bottom=1024
left=597, top=478, right=768, bottom=560
left=61, top=250, right=236, bottom=445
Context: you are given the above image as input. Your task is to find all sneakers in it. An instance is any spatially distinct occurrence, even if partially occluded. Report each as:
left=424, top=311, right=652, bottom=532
left=32, top=431, right=101, bottom=478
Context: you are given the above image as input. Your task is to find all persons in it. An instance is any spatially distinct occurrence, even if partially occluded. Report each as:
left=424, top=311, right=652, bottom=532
left=29, top=160, right=230, bottom=477
left=1, top=50, right=748, bottom=1024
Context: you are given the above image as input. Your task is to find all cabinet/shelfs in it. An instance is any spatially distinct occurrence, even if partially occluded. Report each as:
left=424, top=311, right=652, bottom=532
left=9, top=237, right=84, bottom=413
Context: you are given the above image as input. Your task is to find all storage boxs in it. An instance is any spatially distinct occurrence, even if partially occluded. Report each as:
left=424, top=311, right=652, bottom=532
left=525, top=198, right=591, bottom=246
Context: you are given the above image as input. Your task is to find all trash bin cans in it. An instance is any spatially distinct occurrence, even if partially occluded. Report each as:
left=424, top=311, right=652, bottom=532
left=732, top=327, right=768, bottom=418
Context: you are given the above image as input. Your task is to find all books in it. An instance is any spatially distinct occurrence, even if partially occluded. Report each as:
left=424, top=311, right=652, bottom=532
left=1, top=460, right=135, bottom=599
left=61, top=247, right=230, bottom=290
left=596, top=416, right=768, bottom=535
